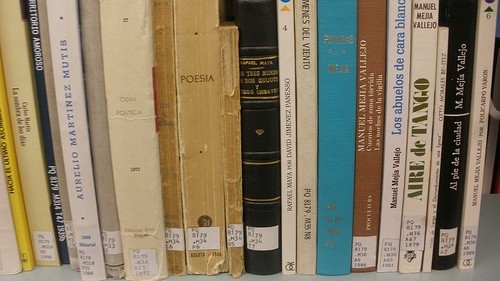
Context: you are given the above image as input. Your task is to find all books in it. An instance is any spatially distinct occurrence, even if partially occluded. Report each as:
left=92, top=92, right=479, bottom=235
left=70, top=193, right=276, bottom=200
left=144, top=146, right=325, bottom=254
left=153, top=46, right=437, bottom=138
left=0, top=0, right=500, bottom=281
